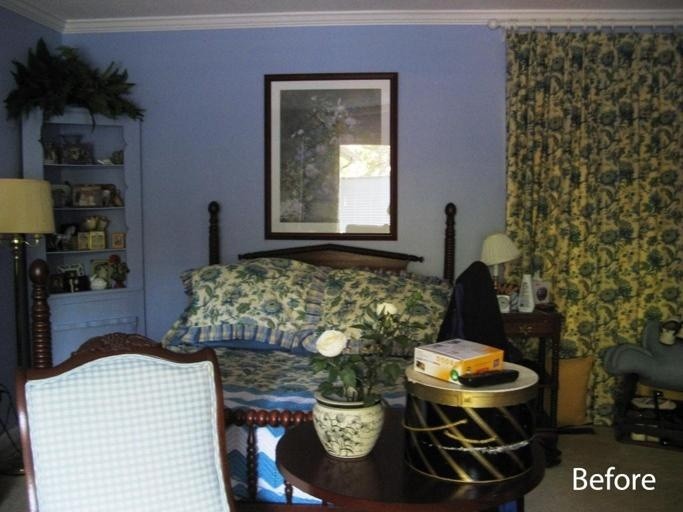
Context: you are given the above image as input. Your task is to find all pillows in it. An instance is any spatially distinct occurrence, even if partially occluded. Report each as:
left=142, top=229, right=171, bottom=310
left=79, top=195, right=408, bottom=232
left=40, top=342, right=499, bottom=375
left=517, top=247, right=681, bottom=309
left=163, top=257, right=315, bottom=353
left=302, top=264, right=454, bottom=356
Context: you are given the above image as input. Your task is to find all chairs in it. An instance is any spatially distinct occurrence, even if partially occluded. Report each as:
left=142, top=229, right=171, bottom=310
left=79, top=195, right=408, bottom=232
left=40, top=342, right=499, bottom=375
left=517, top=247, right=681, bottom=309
left=603, top=317, right=681, bottom=447
left=16, top=332, right=234, bottom=511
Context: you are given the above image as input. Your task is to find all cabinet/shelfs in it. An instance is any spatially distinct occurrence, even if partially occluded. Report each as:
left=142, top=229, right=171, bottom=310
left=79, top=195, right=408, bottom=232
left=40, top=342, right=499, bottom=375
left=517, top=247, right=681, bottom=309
left=24, top=108, right=145, bottom=365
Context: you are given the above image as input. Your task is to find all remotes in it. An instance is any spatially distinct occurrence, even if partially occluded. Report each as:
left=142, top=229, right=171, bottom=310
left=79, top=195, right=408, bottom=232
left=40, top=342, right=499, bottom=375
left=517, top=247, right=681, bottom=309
left=459, top=369, right=517, bottom=386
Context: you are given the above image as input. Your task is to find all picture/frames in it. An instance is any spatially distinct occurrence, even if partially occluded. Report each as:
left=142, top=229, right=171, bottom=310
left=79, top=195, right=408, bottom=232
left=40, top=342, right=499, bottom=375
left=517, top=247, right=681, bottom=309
left=266, top=73, right=400, bottom=241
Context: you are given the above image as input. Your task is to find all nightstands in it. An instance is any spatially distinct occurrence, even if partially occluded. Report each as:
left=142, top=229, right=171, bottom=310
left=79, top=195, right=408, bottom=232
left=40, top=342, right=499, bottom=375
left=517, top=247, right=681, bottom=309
left=502, top=308, right=561, bottom=430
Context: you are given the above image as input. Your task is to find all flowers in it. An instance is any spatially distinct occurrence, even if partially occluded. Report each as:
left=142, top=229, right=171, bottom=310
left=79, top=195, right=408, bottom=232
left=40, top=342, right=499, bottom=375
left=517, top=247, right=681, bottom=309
left=310, top=293, right=419, bottom=398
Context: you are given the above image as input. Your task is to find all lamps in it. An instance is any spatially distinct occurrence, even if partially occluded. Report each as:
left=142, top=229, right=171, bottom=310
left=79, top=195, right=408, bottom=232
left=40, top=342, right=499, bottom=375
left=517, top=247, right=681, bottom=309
left=480, top=234, right=520, bottom=296
left=0, top=178, right=57, bottom=367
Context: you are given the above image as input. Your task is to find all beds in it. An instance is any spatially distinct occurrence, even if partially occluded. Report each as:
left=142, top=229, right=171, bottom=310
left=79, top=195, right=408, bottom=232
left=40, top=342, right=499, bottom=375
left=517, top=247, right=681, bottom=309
left=163, top=202, right=457, bottom=503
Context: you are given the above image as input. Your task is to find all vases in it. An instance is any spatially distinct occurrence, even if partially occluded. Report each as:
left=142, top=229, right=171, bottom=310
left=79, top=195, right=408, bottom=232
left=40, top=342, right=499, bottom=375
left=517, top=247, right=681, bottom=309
left=311, top=393, right=384, bottom=459
